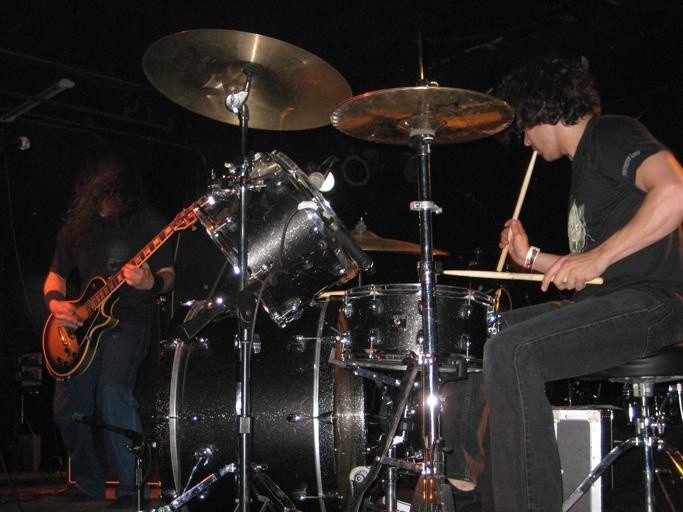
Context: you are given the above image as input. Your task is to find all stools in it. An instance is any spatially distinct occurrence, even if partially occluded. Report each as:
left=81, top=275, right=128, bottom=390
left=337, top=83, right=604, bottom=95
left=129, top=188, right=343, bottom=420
left=560, top=348, right=683, bottom=511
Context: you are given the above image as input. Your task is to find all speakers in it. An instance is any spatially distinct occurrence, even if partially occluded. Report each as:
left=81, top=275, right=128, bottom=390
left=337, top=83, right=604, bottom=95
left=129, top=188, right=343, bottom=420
left=552, top=405, right=683, bottom=512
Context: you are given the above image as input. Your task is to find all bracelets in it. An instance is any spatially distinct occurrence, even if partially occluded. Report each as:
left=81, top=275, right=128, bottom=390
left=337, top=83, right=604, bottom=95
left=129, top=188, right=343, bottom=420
left=522, top=246, right=541, bottom=271
left=44, top=290, right=64, bottom=309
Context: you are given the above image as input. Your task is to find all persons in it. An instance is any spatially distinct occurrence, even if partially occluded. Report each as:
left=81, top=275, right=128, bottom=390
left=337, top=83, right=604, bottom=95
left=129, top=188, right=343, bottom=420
left=382, top=54, right=683, bottom=511
left=43, top=144, right=177, bottom=512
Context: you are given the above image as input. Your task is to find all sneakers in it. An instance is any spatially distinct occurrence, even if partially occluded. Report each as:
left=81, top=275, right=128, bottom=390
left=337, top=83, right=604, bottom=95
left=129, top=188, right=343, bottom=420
left=47, top=488, right=105, bottom=502
left=108, top=496, right=149, bottom=511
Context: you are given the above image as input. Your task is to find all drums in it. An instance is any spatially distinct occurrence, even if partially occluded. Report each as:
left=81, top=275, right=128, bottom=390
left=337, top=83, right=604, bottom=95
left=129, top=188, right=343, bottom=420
left=192, top=149, right=373, bottom=327
left=342, top=283, right=503, bottom=375
left=168, top=294, right=369, bottom=512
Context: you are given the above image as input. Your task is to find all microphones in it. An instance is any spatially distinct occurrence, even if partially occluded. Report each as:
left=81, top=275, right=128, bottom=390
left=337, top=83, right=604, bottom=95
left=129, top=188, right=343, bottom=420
left=1, top=135, right=31, bottom=153
left=309, top=154, right=337, bottom=188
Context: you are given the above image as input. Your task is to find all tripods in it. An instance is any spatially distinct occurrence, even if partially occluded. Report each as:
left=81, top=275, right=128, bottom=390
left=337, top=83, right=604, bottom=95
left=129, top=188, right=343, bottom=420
left=152, top=103, right=304, bottom=512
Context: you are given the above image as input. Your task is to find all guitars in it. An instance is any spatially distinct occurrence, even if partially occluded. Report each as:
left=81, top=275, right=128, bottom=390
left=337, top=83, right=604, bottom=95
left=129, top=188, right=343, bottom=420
left=42, top=195, right=208, bottom=382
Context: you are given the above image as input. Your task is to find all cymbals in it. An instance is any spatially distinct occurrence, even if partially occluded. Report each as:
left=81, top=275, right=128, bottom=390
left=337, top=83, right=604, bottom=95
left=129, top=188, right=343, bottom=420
left=142, top=29, right=354, bottom=131
left=329, top=85, right=516, bottom=145
left=349, top=226, right=447, bottom=255
